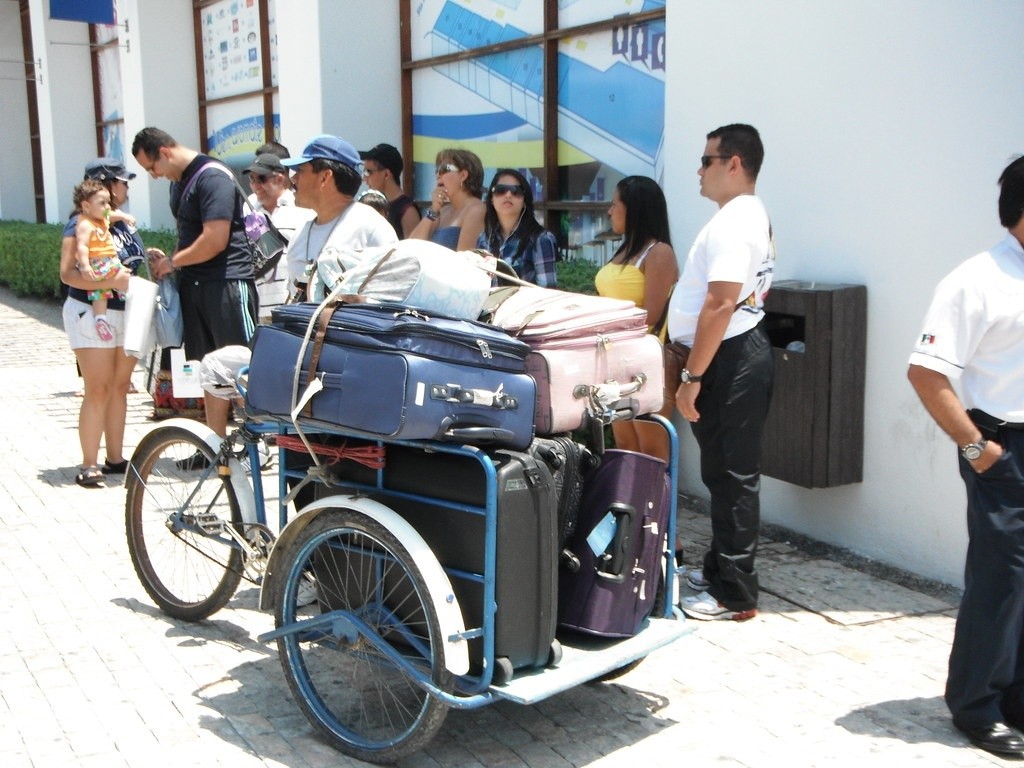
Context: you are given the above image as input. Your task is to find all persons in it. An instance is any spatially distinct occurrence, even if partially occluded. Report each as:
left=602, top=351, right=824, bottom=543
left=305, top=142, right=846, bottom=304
left=594, top=176, right=691, bottom=461
left=668, top=123, right=776, bottom=621
left=906, top=157, right=1024, bottom=756
left=279, top=135, right=398, bottom=303
left=408, top=148, right=486, bottom=253
left=73, top=179, right=136, bottom=341
left=242, top=142, right=317, bottom=324
left=59, top=158, right=145, bottom=484
left=475, top=169, right=557, bottom=289
left=357, top=143, right=422, bottom=240
left=130, top=127, right=259, bottom=469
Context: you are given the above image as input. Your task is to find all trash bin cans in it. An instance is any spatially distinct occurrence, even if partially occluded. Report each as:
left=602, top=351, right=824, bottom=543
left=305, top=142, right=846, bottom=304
left=758, top=280, right=867, bottom=488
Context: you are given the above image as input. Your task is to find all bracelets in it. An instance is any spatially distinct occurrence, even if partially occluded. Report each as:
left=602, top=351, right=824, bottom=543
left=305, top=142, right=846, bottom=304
left=167, top=256, right=176, bottom=271
left=426, top=209, right=437, bottom=222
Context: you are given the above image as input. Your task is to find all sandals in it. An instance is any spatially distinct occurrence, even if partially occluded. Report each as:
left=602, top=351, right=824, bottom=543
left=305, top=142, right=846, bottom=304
left=76, top=466, right=106, bottom=482
left=101, top=457, right=129, bottom=474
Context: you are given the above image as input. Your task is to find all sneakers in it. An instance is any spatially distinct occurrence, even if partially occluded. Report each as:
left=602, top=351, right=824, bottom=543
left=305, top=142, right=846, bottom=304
left=688, top=569, right=716, bottom=590
left=680, top=590, right=755, bottom=620
left=241, top=438, right=272, bottom=475
left=177, top=442, right=233, bottom=468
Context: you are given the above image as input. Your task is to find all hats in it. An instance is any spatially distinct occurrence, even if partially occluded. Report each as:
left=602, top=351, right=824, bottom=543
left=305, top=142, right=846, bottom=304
left=356, top=143, right=405, bottom=172
left=242, top=154, right=288, bottom=174
left=280, top=134, right=365, bottom=178
left=83, top=158, right=136, bottom=183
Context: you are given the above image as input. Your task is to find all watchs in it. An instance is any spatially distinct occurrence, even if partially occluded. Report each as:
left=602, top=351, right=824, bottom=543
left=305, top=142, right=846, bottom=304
left=680, top=369, right=702, bottom=384
left=962, top=438, right=988, bottom=461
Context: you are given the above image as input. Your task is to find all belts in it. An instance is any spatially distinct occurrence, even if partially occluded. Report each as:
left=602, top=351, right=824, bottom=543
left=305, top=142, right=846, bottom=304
left=964, top=410, right=1024, bottom=433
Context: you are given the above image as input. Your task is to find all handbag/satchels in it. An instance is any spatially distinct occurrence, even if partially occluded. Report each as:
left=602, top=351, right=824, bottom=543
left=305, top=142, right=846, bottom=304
left=122, top=252, right=186, bottom=359
left=658, top=342, right=692, bottom=398
left=241, top=213, right=288, bottom=280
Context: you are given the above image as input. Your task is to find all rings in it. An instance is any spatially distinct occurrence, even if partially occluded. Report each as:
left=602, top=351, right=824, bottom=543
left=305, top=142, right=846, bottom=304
left=439, top=194, right=441, bottom=198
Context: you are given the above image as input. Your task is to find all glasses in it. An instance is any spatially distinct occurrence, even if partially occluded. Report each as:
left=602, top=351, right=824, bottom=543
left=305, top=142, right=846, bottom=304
left=146, top=148, right=161, bottom=175
left=360, top=168, right=386, bottom=178
left=249, top=174, right=277, bottom=184
left=490, top=183, right=524, bottom=199
left=701, top=155, right=733, bottom=168
left=435, top=164, right=461, bottom=175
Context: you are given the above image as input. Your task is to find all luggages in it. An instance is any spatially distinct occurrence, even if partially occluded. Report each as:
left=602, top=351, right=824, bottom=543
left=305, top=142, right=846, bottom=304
left=246, top=238, right=674, bottom=683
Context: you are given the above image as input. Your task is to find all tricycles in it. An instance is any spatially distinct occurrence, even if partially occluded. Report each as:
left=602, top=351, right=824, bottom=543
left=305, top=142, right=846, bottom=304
left=122, top=362, right=689, bottom=766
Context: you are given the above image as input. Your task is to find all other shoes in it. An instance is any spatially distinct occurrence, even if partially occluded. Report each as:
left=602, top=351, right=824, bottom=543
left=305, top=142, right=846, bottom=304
left=97, top=318, right=113, bottom=342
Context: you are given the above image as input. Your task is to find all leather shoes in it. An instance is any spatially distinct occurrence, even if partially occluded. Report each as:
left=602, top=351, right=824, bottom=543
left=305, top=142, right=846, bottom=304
left=953, top=712, right=1024, bottom=753
